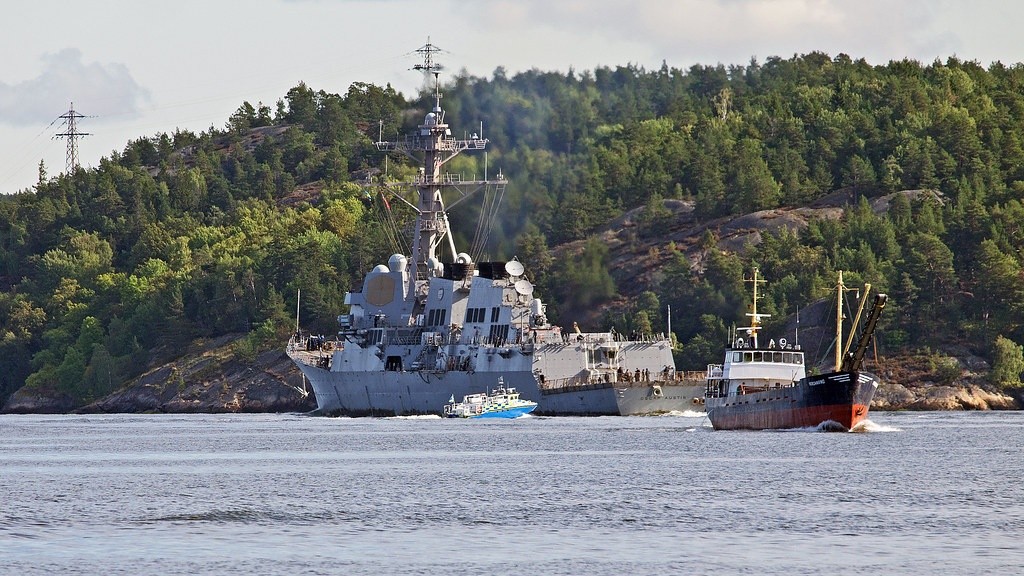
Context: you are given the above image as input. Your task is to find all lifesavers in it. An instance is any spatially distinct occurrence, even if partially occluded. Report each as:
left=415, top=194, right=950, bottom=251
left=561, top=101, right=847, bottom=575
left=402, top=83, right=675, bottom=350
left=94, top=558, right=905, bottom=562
left=537, top=409, right=557, bottom=416
left=577, top=334, right=584, bottom=340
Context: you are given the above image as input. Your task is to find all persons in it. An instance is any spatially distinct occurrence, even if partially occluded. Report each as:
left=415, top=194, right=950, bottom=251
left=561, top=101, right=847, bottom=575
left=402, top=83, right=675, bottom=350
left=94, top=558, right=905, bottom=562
left=765, top=381, right=770, bottom=388
left=533, top=330, right=537, bottom=341
left=617, top=366, right=651, bottom=383
left=599, top=375, right=602, bottom=384
left=660, top=364, right=674, bottom=379
left=742, top=382, right=747, bottom=394
left=604, top=372, right=609, bottom=382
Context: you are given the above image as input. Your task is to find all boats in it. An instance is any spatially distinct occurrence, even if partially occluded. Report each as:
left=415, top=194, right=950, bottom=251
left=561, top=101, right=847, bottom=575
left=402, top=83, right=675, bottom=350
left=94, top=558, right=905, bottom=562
left=284, top=62, right=706, bottom=417
left=445, top=374, right=539, bottom=420
left=706, top=265, right=890, bottom=431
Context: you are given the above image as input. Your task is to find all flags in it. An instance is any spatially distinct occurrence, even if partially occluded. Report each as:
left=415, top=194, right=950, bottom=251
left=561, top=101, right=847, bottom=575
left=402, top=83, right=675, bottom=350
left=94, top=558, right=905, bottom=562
left=449, top=395, right=455, bottom=403
left=498, top=376, right=504, bottom=383
